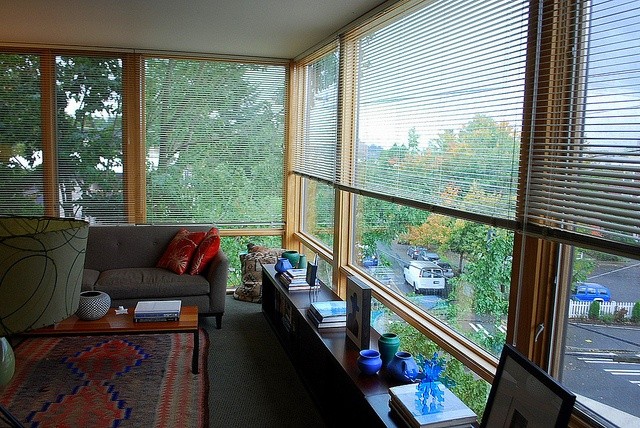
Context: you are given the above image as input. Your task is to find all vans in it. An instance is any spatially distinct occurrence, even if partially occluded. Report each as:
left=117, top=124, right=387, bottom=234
left=404, top=261, right=446, bottom=293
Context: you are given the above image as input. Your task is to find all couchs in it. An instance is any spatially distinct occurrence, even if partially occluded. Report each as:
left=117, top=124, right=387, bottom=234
left=81, top=225, right=228, bottom=330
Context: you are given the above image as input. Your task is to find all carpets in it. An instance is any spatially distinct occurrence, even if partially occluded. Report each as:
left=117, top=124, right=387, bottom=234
left=1, top=327, right=210, bottom=428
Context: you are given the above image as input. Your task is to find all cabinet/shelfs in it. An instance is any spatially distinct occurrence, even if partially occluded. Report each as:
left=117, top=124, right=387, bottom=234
left=261, top=264, right=343, bottom=365
left=295, top=308, right=474, bottom=428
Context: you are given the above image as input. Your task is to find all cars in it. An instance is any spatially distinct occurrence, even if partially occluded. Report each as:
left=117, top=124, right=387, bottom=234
left=361, top=251, right=378, bottom=267
left=417, top=251, right=439, bottom=261
left=434, top=261, right=454, bottom=278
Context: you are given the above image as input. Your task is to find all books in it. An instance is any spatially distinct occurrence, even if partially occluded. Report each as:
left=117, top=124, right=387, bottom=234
left=345, top=275, right=371, bottom=351
left=280, top=278, right=320, bottom=291
left=307, top=308, right=347, bottom=329
left=280, top=274, right=320, bottom=286
left=388, top=381, right=478, bottom=428
left=310, top=300, right=347, bottom=323
left=286, top=268, right=308, bottom=279
left=134, top=300, right=181, bottom=318
left=283, top=272, right=320, bottom=284
left=133, top=317, right=179, bottom=323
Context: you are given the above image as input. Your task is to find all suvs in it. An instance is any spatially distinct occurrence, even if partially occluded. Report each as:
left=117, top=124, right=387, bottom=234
left=568, top=283, right=611, bottom=303
left=406, top=246, right=427, bottom=259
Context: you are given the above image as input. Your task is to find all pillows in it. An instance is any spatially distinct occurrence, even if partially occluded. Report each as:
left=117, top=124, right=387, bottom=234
left=232, top=252, right=277, bottom=304
left=156, top=227, right=207, bottom=277
left=190, top=227, right=221, bottom=276
left=246, top=242, right=288, bottom=258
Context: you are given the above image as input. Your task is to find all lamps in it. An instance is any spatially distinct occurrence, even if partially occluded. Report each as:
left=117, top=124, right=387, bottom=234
left=0, top=215, right=90, bottom=391
